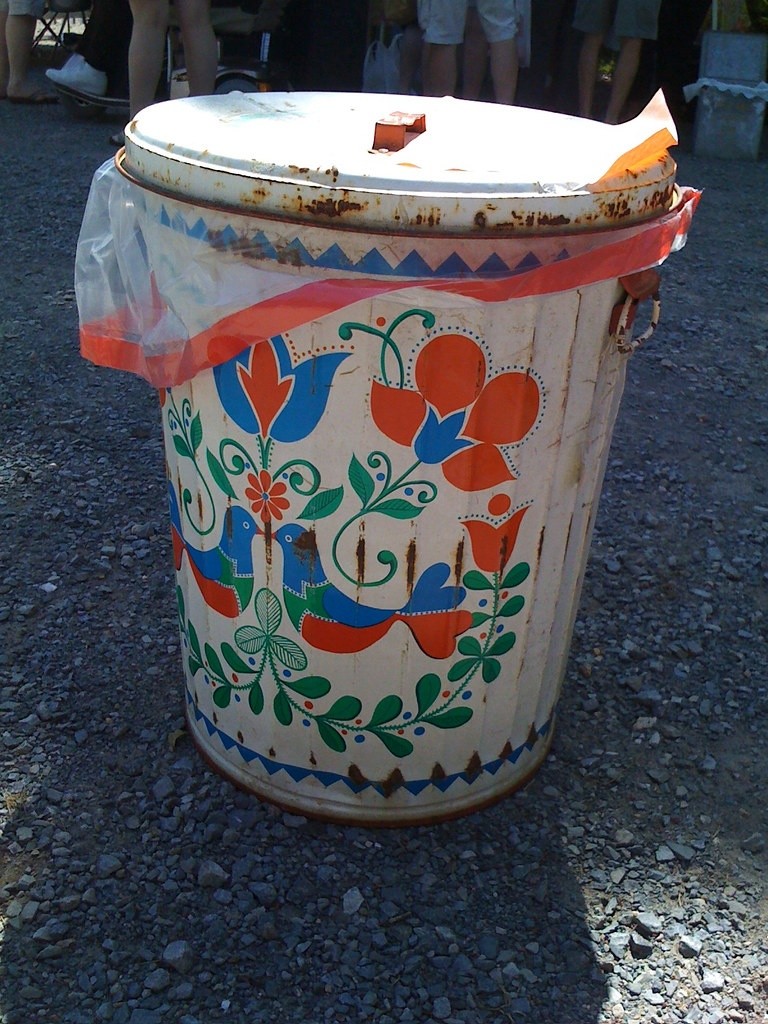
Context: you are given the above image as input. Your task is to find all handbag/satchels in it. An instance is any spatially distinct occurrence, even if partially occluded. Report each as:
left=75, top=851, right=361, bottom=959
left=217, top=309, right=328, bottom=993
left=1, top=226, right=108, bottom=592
left=363, top=34, right=416, bottom=95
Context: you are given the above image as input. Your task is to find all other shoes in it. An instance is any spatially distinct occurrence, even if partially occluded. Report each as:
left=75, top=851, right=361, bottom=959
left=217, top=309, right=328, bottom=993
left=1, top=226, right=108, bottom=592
left=109, top=132, right=125, bottom=145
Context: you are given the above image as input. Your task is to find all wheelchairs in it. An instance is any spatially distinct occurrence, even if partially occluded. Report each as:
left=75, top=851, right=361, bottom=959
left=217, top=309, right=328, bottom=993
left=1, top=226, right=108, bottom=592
left=54, top=0, right=275, bottom=118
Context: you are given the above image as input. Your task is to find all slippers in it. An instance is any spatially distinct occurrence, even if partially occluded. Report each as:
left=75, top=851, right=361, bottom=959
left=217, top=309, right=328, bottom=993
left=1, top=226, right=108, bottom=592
left=0, top=87, right=61, bottom=103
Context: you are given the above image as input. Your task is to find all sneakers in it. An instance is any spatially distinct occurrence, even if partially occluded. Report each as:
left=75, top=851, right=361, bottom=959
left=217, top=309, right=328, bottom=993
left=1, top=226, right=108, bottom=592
left=45, top=61, right=108, bottom=97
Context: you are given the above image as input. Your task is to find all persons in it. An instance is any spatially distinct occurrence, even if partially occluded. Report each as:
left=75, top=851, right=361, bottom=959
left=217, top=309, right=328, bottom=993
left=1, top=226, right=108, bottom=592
left=46, top=0, right=130, bottom=97
left=395, top=0, right=519, bottom=105
left=570, top=0, right=662, bottom=125
left=0, top=0, right=60, bottom=103
left=110, top=0, right=218, bottom=146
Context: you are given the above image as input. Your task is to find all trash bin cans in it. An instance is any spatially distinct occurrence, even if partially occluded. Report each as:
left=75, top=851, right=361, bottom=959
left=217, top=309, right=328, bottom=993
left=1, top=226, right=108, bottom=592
left=113, top=89, right=679, bottom=825
